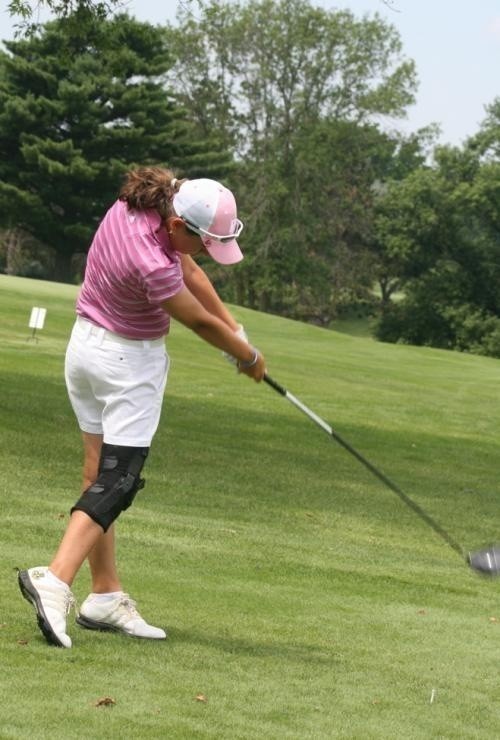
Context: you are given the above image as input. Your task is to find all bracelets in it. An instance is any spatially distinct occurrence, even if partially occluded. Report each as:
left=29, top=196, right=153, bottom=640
left=245, top=349, right=258, bottom=367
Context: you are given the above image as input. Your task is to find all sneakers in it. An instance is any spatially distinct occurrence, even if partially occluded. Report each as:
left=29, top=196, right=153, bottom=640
left=77, top=591, right=166, bottom=640
left=17, top=565, right=76, bottom=649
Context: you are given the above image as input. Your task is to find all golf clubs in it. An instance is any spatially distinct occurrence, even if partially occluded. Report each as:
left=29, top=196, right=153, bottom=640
left=263, top=372, right=500, bottom=573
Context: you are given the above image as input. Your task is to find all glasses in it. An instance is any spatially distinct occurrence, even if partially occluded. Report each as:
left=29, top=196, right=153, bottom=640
left=179, top=218, right=244, bottom=243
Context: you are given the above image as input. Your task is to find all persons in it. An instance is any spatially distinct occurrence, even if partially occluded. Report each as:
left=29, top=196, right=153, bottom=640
left=17, top=164, right=268, bottom=649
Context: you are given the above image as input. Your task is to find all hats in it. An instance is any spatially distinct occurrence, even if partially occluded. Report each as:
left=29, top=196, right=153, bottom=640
left=173, top=176, right=245, bottom=266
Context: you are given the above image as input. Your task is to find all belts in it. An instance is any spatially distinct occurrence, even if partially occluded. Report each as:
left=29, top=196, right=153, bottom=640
left=76, top=315, right=166, bottom=350
